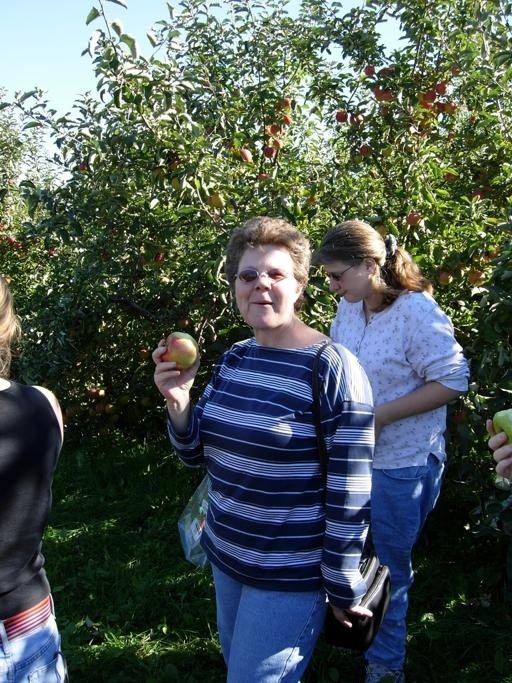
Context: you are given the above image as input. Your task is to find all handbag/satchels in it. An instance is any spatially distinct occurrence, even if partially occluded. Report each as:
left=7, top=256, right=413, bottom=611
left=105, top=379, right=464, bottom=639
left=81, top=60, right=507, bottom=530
left=324, top=341, right=392, bottom=652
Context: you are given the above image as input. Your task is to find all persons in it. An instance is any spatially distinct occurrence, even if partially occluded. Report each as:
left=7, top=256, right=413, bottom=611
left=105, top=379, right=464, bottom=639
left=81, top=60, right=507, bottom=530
left=0, top=274, right=69, bottom=683
left=485, top=419, right=512, bottom=483
left=152, top=215, right=375, bottom=683
left=318, top=219, right=470, bottom=683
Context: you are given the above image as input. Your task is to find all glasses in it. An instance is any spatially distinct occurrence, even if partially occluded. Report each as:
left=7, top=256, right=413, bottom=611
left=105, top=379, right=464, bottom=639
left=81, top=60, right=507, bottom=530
left=234, top=269, right=288, bottom=282
left=324, top=264, right=355, bottom=282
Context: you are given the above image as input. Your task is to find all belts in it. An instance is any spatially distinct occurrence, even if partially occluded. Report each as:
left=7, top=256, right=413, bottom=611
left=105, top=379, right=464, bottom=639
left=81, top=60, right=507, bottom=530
left=0, top=595, right=52, bottom=646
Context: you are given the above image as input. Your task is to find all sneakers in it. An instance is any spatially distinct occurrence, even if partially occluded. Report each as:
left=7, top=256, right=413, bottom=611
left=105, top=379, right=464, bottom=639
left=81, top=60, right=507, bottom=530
left=365, top=664, right=405, bottom=683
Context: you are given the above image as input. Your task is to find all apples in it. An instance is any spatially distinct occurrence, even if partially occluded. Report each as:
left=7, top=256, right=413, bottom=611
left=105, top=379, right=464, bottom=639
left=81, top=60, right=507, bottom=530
left=161, top=331, right=199, bottom=370
left=136, top=348, right=149, bottom=359
left=493, top=407, right=512, bottom=445
left=0, top=65, right=500, bottom=328
left=41, top=378, right=120, bottom=436
